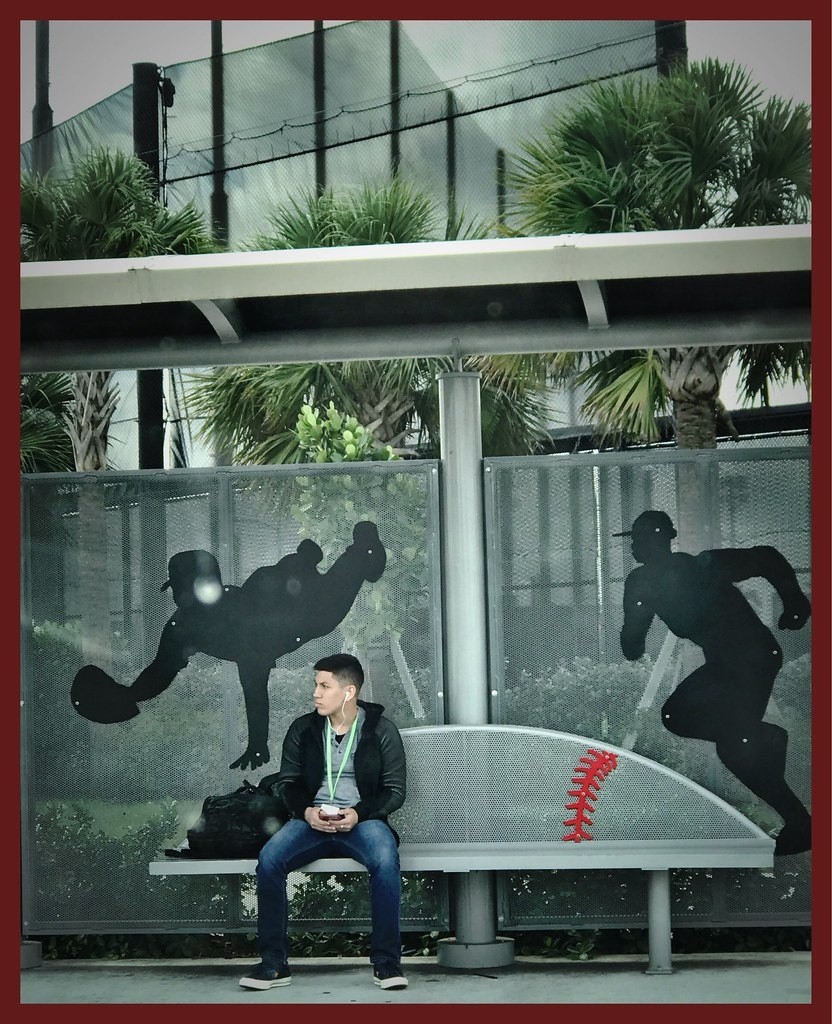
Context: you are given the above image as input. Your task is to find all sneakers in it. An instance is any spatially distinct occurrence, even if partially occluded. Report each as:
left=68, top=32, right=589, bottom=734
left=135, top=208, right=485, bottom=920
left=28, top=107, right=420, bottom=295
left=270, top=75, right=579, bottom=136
left=238, top=962, right=291, bottom=990
left=372, top=962, right=408, bottom=990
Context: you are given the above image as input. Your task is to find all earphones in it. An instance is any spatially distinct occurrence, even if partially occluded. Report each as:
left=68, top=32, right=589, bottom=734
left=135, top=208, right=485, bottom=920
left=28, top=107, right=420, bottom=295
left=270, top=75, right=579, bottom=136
left=345, top=692, right=350, bottom=700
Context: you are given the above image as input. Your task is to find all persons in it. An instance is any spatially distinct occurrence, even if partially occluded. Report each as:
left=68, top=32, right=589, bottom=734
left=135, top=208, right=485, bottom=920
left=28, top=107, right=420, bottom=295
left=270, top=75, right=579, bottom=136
left=238, top=653, right=409, bottom=991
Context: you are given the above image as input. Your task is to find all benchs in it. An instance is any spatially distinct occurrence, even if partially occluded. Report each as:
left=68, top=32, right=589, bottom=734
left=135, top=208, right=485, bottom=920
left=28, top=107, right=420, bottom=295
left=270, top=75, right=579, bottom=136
left=149, top=724, right=777, bottom=975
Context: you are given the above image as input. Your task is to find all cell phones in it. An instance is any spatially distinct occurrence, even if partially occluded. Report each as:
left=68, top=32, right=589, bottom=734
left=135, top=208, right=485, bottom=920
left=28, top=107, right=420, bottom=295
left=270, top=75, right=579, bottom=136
left=319, top=814, right=342, bottom=821
